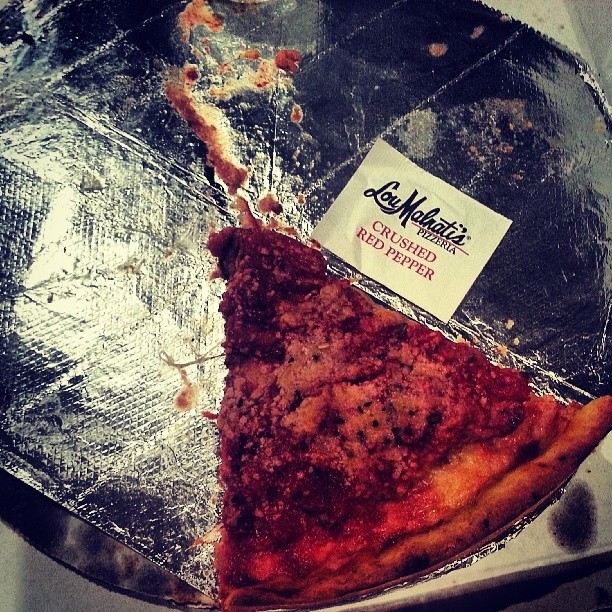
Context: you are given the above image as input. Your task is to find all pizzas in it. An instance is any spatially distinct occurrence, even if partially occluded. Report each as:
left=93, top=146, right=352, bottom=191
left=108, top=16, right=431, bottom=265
left=204, top=227, right=612, bottom=612
